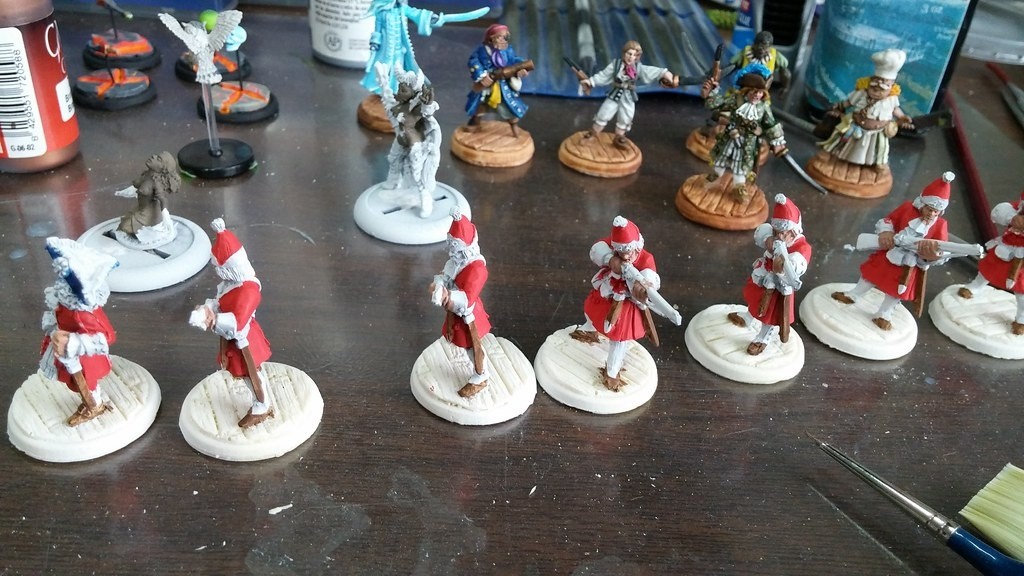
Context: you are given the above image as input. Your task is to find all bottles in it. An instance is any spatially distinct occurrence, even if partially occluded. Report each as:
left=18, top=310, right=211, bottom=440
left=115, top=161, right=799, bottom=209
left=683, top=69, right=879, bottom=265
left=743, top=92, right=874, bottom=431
left=0, top=0, right=80, bottom=173
left=309, top=0, right=376, bottom=69
left=733, top=0, right=806, bottom=88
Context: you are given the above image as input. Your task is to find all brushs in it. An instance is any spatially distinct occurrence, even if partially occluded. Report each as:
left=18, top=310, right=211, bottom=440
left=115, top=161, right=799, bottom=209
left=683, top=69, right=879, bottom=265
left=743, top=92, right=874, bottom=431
left=957, top=460, right=1024, bottom=560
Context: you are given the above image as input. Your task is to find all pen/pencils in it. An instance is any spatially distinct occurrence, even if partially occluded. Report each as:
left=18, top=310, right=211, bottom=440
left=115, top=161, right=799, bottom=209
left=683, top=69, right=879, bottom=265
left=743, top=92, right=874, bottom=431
left=803, top=428, right=1024, bottom=576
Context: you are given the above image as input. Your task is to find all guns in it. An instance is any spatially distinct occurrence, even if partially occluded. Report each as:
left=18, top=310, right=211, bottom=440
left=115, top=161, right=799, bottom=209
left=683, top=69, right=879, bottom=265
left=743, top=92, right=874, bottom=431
left=432, top=281, right=444, bottom=307
left=701, top=43, right=723, bottom=99
left=621, top=260, right=681, bottom=327
left=855, top=233, right=983, bottom=256
left=189, top=297, right=221, bottom=331
left=471, top=59, right=534, bottom=91
left=561, top=56, right=591, bottom=96
left=771, top=239, right=802, bottom=291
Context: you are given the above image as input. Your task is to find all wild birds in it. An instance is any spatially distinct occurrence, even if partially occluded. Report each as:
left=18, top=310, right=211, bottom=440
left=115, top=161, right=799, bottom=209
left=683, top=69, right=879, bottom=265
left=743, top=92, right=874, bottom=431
left=157, top=10, right=242, bottom=85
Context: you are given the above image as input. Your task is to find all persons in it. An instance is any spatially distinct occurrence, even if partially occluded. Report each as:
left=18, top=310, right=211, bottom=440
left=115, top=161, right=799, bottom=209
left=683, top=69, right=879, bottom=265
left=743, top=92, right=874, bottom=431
left=718, top=30, right=792, bottom=100
left=34, top=236, right=123, bottom=428
left=958, top=190, right=1024, bottom=335
left=188, top=217, right=273, bottom=429
left=727, top=192, right=813, bottom=357
left=360, top=0, right=439, bottom=97
left=567, top=214, right=660, bottom=392
left=371, top=62, right=442, bottom=218
left=112, top=149, right=182, bottom=249
left=463, top=24, right=534, bottom=138
left=426, top=206, right=492, bottom=400
left=577, top=39, right=674, bottom=150
left=814, top=47, right=916, bottom=166
left=700, top=62, right=790, bottom=204
left=831, top=169, right=956, bottom=331
